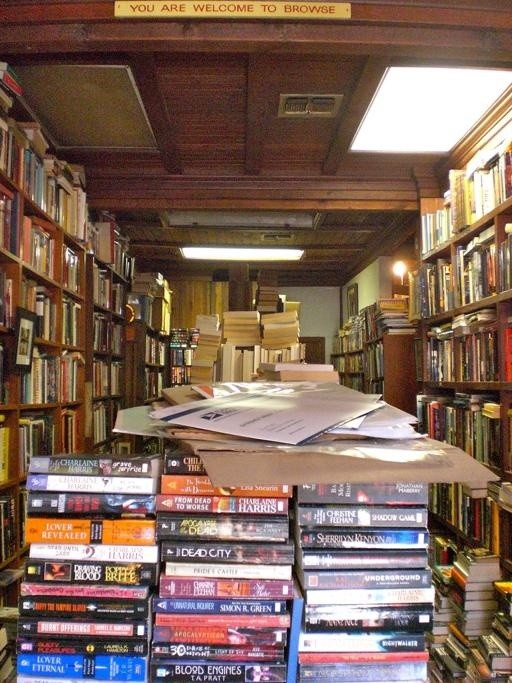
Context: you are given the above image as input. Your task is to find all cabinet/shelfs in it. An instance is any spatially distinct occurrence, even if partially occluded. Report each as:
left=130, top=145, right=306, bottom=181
left=169, top=327, right=200, bottom=388
left=365, top=335, right=416, bottom=416
left=88, top=220, right=131, bottom=451
left=422, top=165, right=512, bottom=575
left=1, top=117, right=87, bottom=683
left=128, top=269, right=172, bottom=406
left=331, top=328, right=364, bottom=392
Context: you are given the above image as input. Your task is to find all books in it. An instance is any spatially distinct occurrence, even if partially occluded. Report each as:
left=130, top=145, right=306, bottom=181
left=420, top=140, right=512, bottom=255
left=422, top=223, right=512, bottom=318
left=0, top=181, right=86, bottom=294
left=148, top=454, right=294, bottom=682
left=417, top=393, right=512, bottom=472
left=430, top=481, right=512, bottom=560
left=94, top=314, right=126, bottom=353
left=128, top=270, right=174, bottom=337
left=286, top=481, right=434, bottom=681
left=1, top=570, right=17, bottom=683
left=429, top=543, right=511, bottom=683
left=0, top=351, right=126, bottom=404
left=94, top=263, right=127, bottom=315
left=332, top=341, right=382, bottom=379
left=169, top=272, right=340, bottom=385
left=0, top=63, right=98, bottom=250
left=1, top=403, right=119, bottom=482
left=142, top=365, right=169, bottom=401
left=82, top=206, right=136, bottom=287
left=16, top=455, right=158, bottom=683
left=0, top=269, right=86, bottom=352
left=341, top=376, right=382, bottom=394
left=142, top=333, right=167, bottom=368
left=330, top=297, right=417, bottom=353
left=423, top=310, right=510, bottom=382
left=1, top=485, right=25, bottom=565
